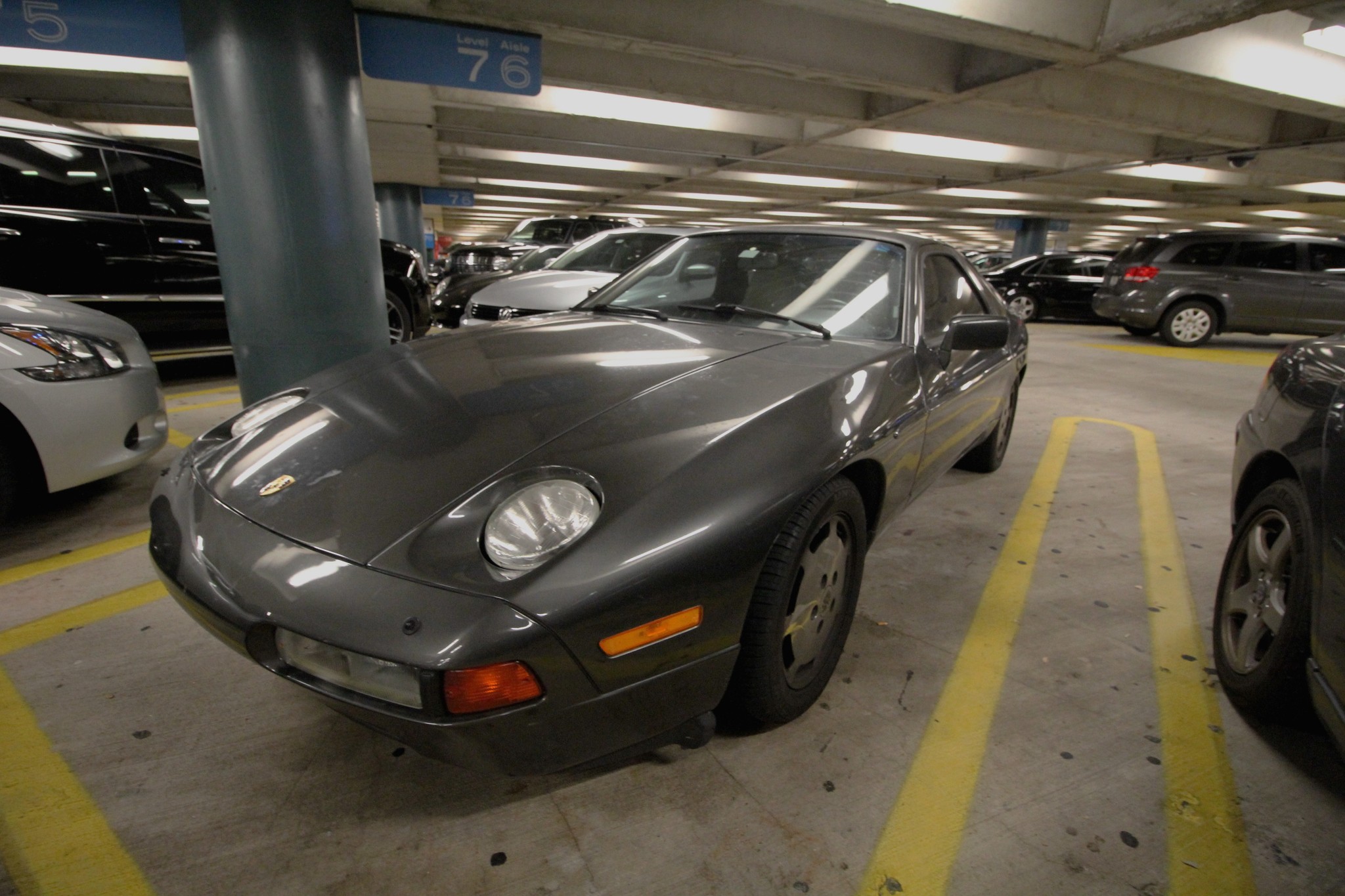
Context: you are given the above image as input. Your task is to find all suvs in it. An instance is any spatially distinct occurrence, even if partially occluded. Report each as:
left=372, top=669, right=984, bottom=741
left=2, top=113, right=434, bottom=371
left=1090, top=224, right=1343, bottom=349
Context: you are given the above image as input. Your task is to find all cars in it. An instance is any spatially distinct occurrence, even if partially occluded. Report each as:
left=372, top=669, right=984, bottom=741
left=977, top=251, right=1123, bottom=323
left=432, top=214, right=717, bottom=336
left=1, top=285, right=169, bottom=529
left=148, top=222, right=1031, bottom=779
left=1206, top=336, right=1345, bottom=753
